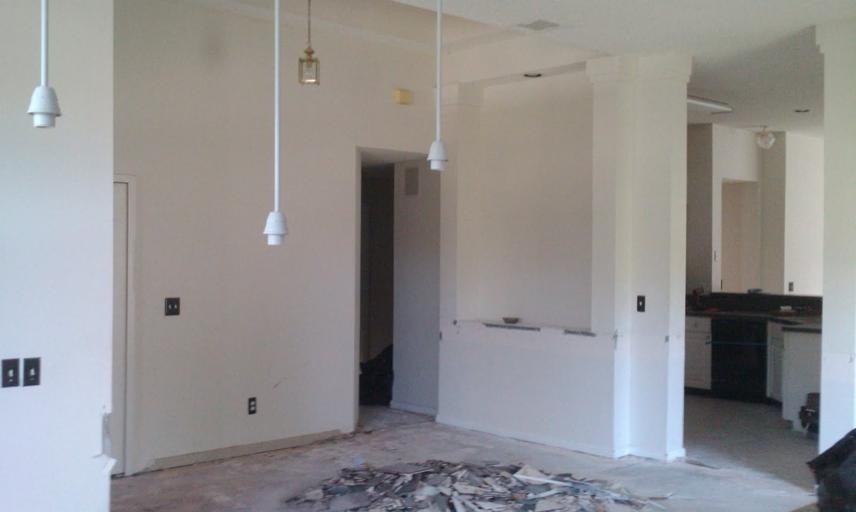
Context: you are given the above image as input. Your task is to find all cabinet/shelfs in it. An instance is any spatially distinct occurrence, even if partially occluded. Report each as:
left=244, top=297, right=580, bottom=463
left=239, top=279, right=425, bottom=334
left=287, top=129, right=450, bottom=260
left=764, top=320, right=820, bottom=422
left=684, top=315, right=711, bottom=390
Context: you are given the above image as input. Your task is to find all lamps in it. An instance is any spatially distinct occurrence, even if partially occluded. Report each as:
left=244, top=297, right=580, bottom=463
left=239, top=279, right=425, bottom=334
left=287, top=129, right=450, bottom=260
left=423, top=0, right=450, bottom=171
left=263, top=0, right=320, bottom=250
left=26, top=0, right=61, bottom=130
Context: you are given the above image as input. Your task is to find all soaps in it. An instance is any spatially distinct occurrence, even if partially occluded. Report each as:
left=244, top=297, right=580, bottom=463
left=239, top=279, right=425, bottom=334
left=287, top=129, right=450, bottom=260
left=779, top=305, right=799, bottom=317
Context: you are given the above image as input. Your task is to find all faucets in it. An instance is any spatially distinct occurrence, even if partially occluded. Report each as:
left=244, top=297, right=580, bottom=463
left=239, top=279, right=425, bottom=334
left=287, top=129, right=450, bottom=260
left=747, top=287, right=763, bottom=307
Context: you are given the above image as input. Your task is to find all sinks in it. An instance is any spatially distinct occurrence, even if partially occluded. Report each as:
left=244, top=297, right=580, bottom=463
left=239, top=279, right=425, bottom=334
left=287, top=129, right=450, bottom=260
left=720, top=305, right=771, bottom=314
